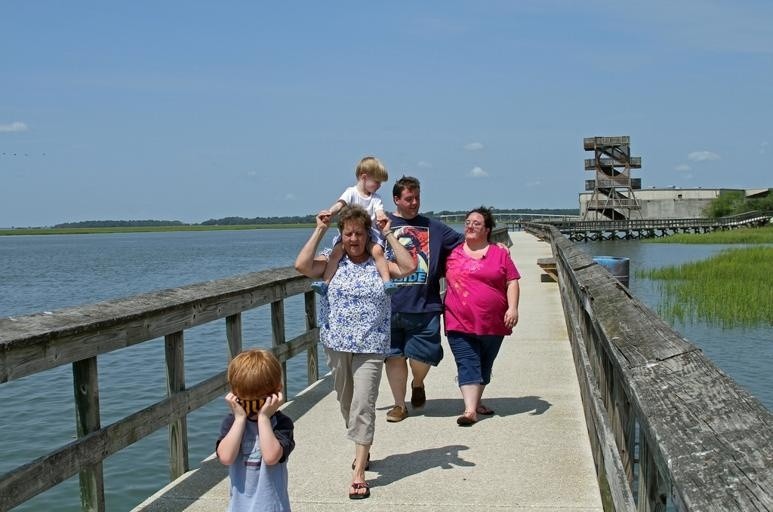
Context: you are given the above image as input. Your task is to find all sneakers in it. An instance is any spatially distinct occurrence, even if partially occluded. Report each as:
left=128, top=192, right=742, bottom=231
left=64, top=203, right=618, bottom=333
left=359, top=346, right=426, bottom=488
left=411, top=379, right=426, bottom=411
left=386, top=404, right=409, bottom=423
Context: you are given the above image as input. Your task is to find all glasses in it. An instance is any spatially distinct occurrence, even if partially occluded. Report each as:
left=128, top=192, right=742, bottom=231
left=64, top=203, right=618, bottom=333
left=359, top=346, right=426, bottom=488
left=465, top=220, right=486, bottom=229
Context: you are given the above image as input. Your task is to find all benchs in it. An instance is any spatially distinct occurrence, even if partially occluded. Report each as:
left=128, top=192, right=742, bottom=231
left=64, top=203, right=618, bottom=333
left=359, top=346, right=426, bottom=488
left=522, top=226, right=544, bottom=241
left=536, top=256, right=557, bottom=282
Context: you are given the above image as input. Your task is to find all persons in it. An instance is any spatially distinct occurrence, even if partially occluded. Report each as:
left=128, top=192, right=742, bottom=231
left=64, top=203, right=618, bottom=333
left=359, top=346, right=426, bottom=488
left=294, top=210, right=417, bottom=499
left=216, top=348, right=296, bottom=512
left=375, top=176, right=511, bottom=427
left=394, top=227, right=428, bottom=273
left=439, top=206, right=521, bottom=425
left=311, top=157, right=397, bottom=297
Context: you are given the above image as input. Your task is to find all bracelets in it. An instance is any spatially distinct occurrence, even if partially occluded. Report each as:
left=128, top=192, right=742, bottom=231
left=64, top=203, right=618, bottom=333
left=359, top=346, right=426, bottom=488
left=383, top=230, right=394, bottom=239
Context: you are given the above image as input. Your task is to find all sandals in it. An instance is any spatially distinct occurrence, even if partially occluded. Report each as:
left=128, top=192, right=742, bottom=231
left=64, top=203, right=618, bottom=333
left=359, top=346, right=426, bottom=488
left=383, top=282, right=398, bottom=292
left=311, top=281, right=327, bottom=295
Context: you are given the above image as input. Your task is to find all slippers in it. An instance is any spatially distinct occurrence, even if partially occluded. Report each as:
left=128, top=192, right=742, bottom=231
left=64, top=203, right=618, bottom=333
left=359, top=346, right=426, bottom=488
left=457, top=403, right=495, bottom=426
left=349, top=482, right=370, bottom=500
left=352, top=450, right=370, bottom=472
